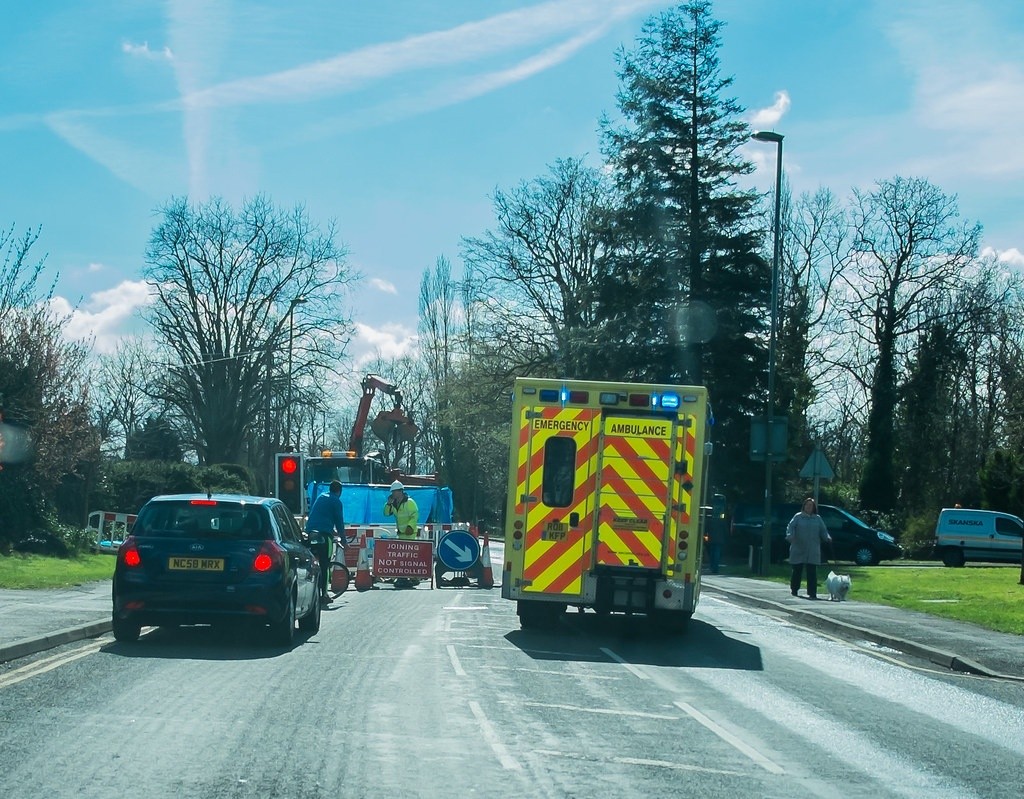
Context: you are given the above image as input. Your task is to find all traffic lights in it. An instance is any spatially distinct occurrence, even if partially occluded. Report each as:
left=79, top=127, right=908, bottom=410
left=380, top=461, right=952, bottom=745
left=276, top=453, right=304, bottom=518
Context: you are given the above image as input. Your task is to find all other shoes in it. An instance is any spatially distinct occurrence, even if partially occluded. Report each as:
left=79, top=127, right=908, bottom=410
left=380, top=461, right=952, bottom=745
left=791, top=587, right=798, bottom=596
left=809, top=593, right=816, bottom=600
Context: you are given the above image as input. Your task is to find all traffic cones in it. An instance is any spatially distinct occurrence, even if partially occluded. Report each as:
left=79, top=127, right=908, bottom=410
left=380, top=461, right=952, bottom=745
left=481, top=533, right=500, bottom=588
left=356, top=534, right=377, bottom=590
left=331, top=540, right=352, bottom=592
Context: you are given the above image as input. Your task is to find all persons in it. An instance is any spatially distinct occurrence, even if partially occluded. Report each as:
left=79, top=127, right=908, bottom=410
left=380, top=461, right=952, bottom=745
left=383, top=481, right=420, bottom=587
left=305, top=480, right=350, bottom=603
left=786, top=498, right=832, bottom=600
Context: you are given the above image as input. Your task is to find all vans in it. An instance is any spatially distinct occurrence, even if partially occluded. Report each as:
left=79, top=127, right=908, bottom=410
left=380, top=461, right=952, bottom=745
left=727, top=504, right=904, bottom=565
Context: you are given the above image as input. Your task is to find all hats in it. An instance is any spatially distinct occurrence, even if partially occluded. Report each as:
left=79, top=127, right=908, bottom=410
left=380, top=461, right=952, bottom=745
left=390, top=479, right=404, bottom=492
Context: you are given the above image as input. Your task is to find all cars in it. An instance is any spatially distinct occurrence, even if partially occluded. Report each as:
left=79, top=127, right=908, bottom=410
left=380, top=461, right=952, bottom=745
left=113, top=490, right=322, bottom=647
left=932, top=504, right=1024, bottom=567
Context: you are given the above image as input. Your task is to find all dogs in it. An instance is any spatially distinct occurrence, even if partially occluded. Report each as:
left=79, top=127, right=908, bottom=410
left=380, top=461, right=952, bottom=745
left=826, top=570, right=852, bottom=602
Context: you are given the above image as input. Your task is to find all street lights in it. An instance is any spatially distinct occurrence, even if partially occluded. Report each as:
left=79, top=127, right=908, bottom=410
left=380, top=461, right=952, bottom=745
left=287, top=298, right=306, bottom=449
left=752, top=131, right=785, bottom=575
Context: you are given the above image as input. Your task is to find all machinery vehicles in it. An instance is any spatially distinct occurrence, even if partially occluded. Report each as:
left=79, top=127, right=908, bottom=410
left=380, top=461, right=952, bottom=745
left=302, top=373, right=478, bottom=549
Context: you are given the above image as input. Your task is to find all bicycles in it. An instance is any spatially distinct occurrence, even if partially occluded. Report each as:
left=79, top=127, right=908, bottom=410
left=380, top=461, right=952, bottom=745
left=302, top=530, right=349, bottom=599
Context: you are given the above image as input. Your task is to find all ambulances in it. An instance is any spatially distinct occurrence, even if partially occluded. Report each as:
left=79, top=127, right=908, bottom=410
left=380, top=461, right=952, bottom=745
left=500, top=377, right=717, bottom=635
